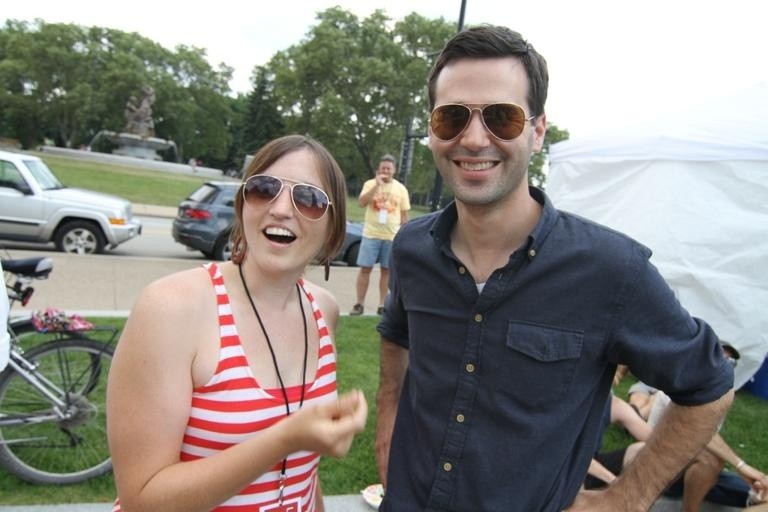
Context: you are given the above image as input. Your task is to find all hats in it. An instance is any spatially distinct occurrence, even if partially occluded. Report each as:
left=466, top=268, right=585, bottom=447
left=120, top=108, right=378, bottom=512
left=720, top=339, right=740, bottom=359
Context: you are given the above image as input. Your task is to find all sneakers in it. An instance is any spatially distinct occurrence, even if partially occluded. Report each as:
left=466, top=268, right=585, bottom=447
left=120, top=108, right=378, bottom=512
left=350, top=304, right=363, bottom=315
left=377, top=305, right=384, bottom=314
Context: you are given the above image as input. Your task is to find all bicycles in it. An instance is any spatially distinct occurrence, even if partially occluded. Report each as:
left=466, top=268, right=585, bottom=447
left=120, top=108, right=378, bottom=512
left=1, top=256, right=114, bottom=488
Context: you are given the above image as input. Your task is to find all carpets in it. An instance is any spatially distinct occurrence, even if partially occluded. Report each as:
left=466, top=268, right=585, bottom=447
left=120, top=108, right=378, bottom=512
left=0, top=491, right=744, bottom=512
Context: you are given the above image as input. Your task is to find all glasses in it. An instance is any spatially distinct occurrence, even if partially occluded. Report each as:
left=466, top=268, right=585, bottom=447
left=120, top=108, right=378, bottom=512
left=240, top=174, right=334, bottom=221
left=728, top=357, right=736, bottom=367
left=428, top=102, right=536, bottom=140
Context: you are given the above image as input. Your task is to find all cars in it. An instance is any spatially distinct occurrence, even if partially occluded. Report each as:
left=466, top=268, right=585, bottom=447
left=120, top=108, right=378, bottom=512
left=0, top=151, right=142, bottom=253
left=172, top=179, right=363, bottom=267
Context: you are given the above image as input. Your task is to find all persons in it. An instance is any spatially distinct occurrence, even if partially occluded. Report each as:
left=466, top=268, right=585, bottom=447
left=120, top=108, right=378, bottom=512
left=104, top=134, right=368, bottom=512
left=582, top=339, right=767, bottom=511
left=372, top=24, right=737, bottom=512
left=348, top=153, right=412, bottom=316
